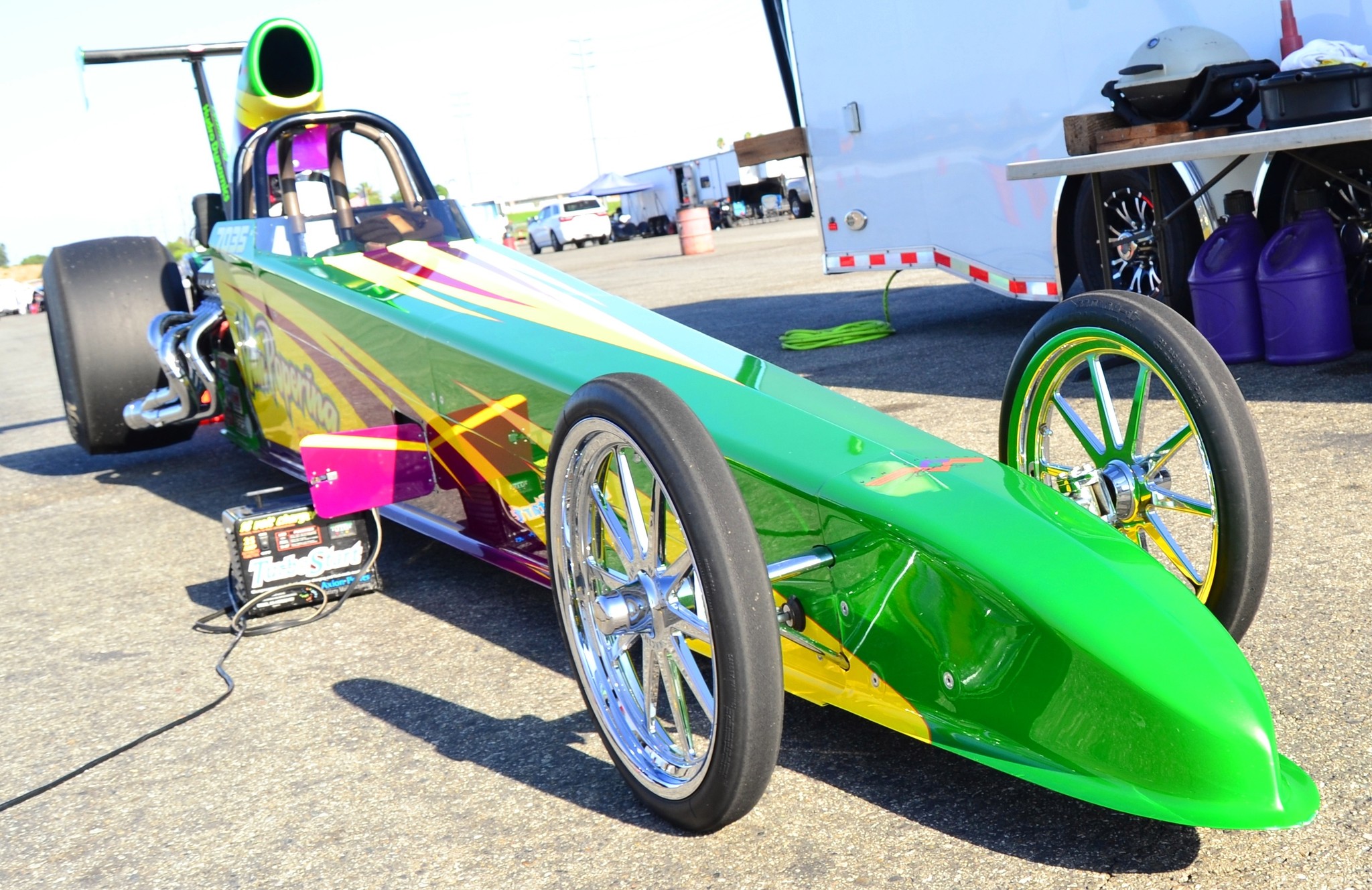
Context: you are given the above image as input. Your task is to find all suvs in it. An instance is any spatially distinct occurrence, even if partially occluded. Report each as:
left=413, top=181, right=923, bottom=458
left=528, top=195, right=612, bottom=256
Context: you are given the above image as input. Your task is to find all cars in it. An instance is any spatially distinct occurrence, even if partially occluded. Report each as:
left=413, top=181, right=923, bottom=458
left=786, top=175, right=814, bottom=220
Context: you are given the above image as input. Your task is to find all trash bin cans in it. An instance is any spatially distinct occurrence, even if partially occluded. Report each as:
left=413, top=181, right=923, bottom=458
left=676, top=203, right=715, bottom=255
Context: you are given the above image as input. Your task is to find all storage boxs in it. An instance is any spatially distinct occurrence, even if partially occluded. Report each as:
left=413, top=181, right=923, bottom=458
left=1255, top=63, right=1372, bottom=134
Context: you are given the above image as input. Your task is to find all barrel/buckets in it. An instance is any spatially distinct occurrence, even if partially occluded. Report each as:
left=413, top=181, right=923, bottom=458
left=676, top=205, right=715, bottom=255
left=1187, top=189, right=1271, bottom=365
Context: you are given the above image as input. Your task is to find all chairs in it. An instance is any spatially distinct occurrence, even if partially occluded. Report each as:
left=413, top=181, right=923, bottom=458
left=731, top=193, right=783, bottom=228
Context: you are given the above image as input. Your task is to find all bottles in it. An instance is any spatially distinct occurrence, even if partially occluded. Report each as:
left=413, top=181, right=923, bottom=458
left=1256, top=187, right=1353, bottom=367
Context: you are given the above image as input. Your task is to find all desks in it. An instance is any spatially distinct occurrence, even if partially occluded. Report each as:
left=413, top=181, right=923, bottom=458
left=1001, top=115, right=1372, bottom=312
